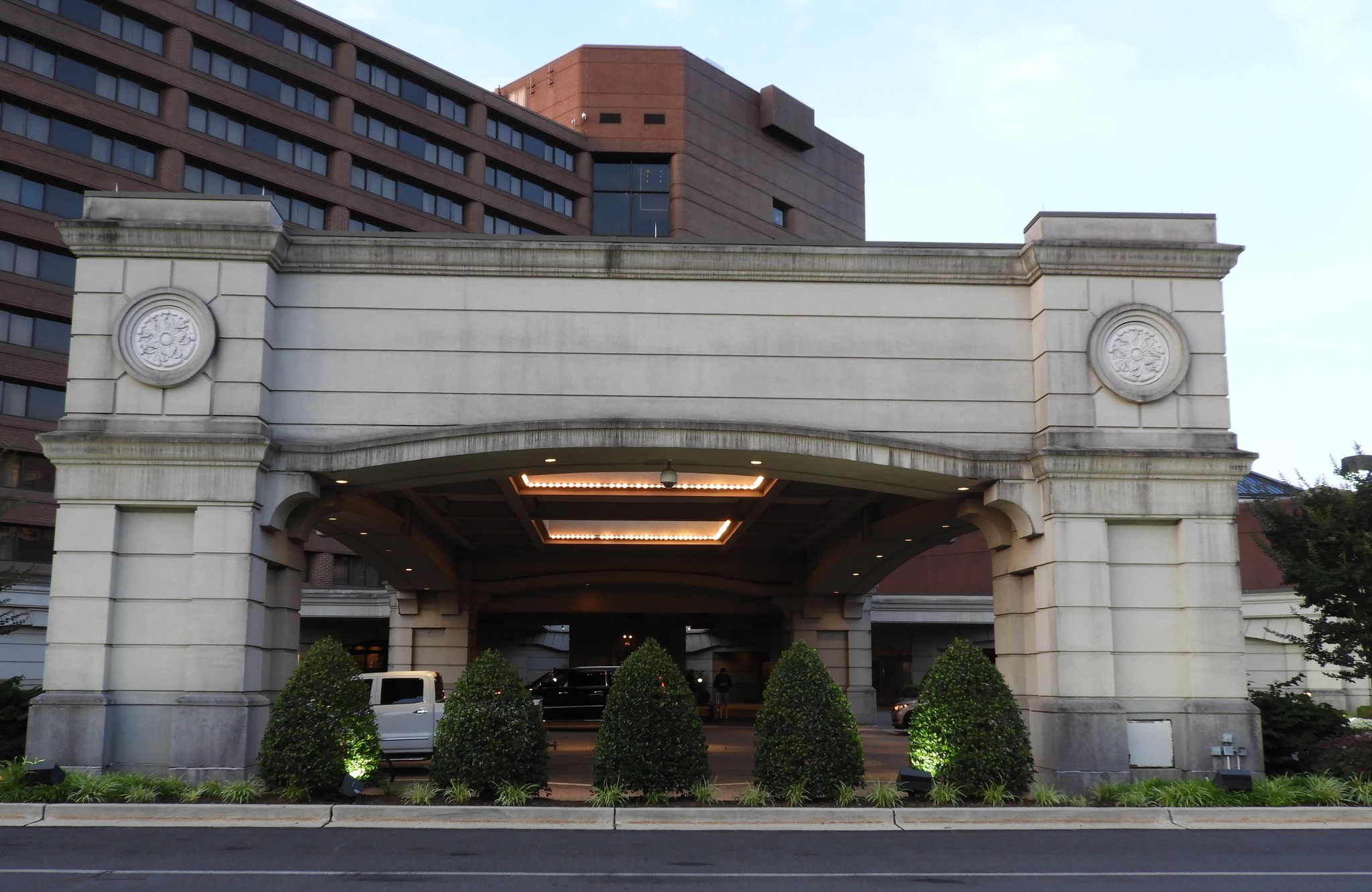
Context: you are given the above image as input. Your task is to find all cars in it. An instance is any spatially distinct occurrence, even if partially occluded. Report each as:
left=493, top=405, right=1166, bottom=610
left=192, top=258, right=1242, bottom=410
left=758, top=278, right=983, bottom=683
left=891, top=698, right=926, bottom=729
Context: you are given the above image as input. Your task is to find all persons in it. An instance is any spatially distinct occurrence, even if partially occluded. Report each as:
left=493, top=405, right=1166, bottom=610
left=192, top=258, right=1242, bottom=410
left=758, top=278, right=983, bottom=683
left=559, top=675, right=575, bottom=687
left=713, top=667, right=732, bottom=720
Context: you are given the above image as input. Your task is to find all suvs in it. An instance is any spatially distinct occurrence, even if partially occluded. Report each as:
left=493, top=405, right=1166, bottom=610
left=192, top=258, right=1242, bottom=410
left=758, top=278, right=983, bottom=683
left=496, top=666, right=624, bottom=721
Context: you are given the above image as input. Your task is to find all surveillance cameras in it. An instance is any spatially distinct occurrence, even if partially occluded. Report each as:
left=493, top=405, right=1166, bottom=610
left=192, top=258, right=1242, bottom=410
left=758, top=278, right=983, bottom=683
left=659, top=467, right=678, bottom=488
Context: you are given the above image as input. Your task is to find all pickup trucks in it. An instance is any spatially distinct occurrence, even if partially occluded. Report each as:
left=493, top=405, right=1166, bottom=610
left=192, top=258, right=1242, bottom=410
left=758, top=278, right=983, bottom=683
left=310, top=671, right=558, bottom=785
